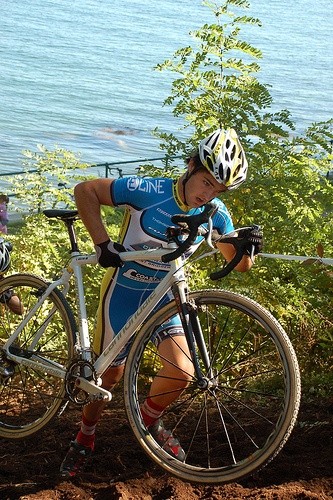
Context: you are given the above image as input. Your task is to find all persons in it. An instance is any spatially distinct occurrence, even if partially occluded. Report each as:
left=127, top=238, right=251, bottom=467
left=0, top=191, right=25, bottom=315
left=59, top=127, right=262, bottom=479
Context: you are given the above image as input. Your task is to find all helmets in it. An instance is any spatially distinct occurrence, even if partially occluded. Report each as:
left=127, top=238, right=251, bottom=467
left=199, top=128, right=248, bottom=190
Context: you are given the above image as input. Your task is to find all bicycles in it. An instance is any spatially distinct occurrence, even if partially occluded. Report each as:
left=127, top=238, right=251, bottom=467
left=0, top=202, right=302, bottom=486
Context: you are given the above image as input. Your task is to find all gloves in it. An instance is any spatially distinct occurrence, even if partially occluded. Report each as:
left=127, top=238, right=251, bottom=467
left=94, top=239, right=127, bottom=268
left=238, top=227, right=263, bottom=255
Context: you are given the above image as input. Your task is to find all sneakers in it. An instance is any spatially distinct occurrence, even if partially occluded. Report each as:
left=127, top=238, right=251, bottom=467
left=58, top=436, right=92, bottom=481
left=145, top=417, right=186, bottom=462
